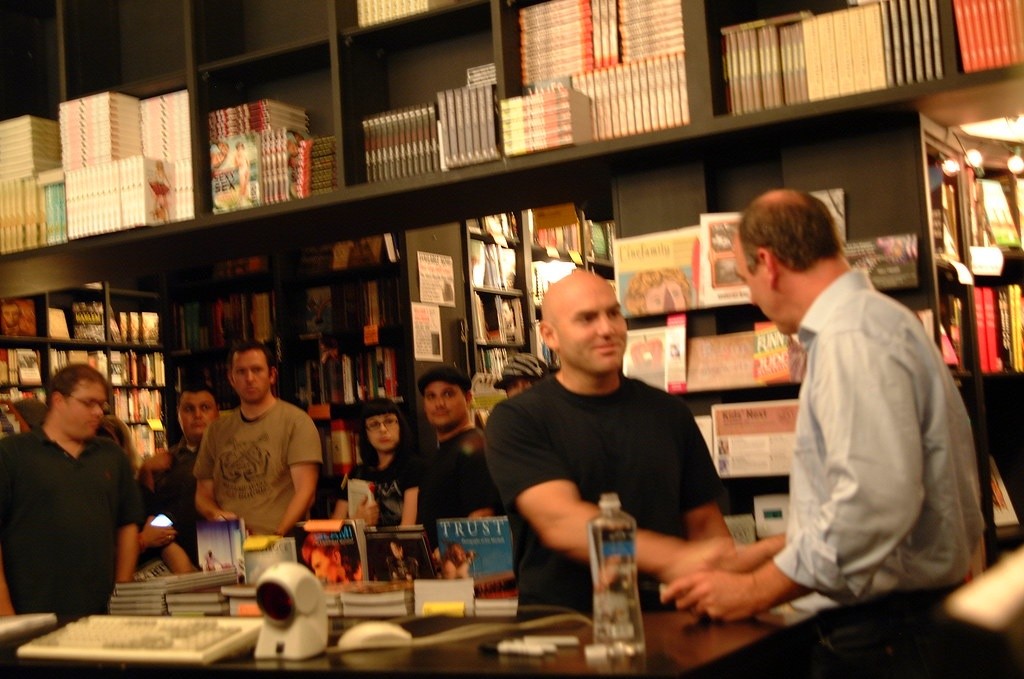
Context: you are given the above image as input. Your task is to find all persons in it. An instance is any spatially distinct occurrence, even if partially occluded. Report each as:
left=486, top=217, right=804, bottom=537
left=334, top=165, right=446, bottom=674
left=148, top=159, right=173, bottom=224
left=0, top=338, right=560, bottom=624
left=301, top=531, right=363, bottom=585
left=658, top=187, right=987, bottom=617
left=668, top=343, right=683, bottom=384
left=386, top=539, right=420, bottom=582
left=210, top=142, right=230, bottom=172
left=234, top=142, right=252, bottom=208
left=428, top=543, right=457, bottom=579
left=445, top=541, right=475, bottom=579
left=205, top=550, right=217, bottom=571
left=482, top=269, right=742, bottom=615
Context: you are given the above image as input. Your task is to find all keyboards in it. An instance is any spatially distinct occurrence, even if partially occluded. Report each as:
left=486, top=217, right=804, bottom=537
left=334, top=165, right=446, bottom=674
left=17, top=616, right=264, bottom=665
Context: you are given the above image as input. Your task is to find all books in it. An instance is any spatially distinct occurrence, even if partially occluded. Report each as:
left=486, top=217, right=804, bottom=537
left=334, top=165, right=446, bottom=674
left=0, top=0, right=1024, bottom=619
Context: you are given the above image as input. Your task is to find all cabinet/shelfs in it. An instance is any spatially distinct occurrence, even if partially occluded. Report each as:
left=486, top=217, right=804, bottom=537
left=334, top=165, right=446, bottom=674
left=0, top=1, right=1022, bottom=571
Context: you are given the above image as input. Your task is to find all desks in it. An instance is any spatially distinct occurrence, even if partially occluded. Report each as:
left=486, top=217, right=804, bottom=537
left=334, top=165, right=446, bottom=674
left=0, top=612, right=784, bottom=679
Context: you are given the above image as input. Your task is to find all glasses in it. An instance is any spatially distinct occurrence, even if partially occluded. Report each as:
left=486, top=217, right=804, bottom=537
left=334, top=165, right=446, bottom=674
left=366, top=419, right=398, bottom=431
left=66, top=393, right=110, bottom=410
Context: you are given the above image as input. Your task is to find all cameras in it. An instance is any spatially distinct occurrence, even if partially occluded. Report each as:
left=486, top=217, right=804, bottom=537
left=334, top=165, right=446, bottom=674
left=150, top=514, right=172, bottom=529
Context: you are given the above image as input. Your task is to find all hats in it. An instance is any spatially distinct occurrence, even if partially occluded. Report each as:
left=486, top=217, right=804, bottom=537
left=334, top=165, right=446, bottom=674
left=494, top=354, right=547, bottom=388
left=418, top=367, right=471, bottom=396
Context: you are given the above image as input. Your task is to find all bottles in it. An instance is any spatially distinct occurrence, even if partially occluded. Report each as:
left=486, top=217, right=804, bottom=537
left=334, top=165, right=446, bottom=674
left=587, top=494, right=646, bottom=653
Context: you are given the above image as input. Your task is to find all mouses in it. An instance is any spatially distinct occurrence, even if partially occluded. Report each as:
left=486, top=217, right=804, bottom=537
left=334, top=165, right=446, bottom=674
left=336, top=621, right=412, bottom=651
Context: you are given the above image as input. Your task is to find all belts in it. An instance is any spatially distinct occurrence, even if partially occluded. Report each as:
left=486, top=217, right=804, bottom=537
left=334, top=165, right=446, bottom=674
left=813, top=583, right=962, bottom=639
